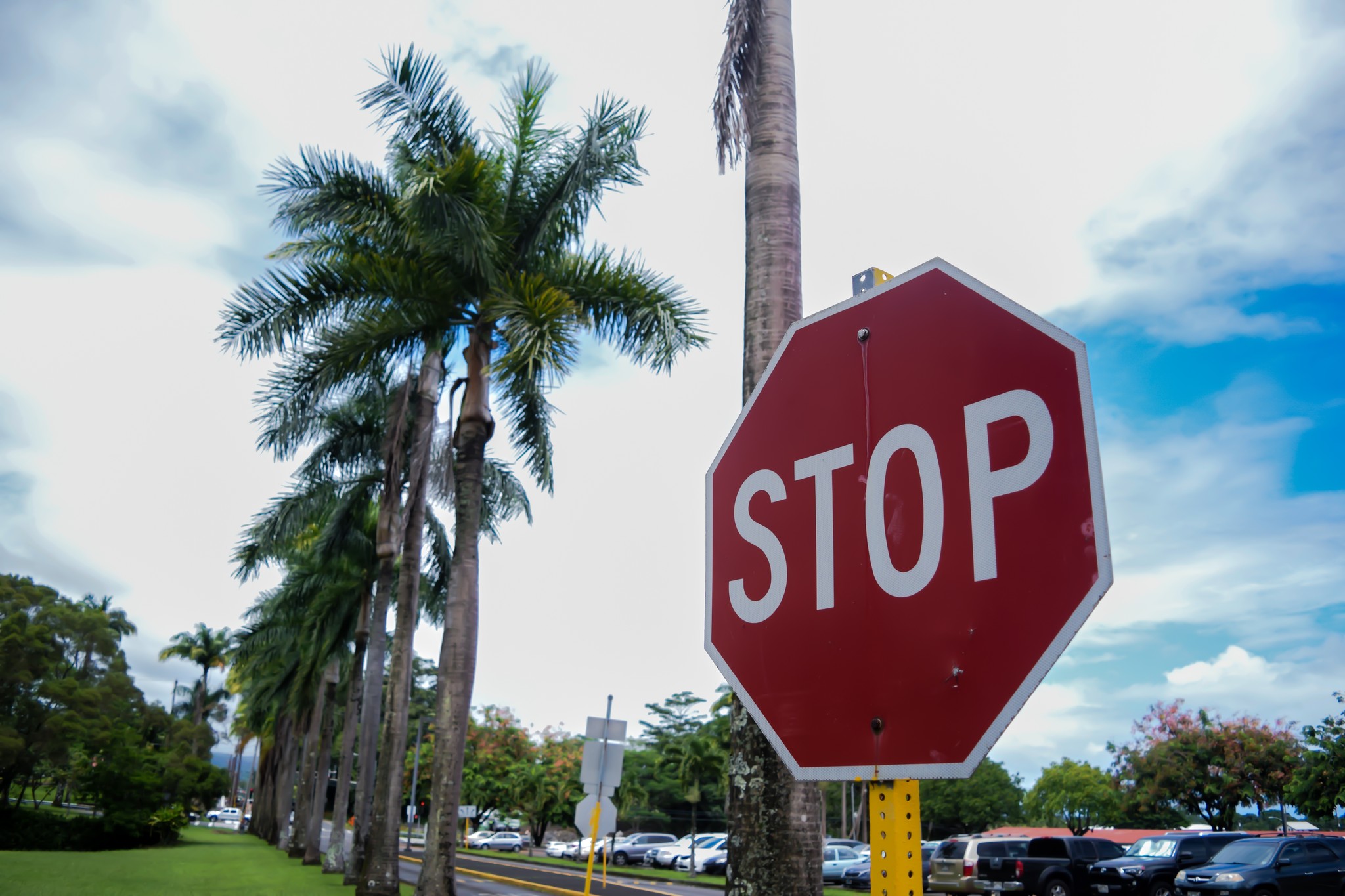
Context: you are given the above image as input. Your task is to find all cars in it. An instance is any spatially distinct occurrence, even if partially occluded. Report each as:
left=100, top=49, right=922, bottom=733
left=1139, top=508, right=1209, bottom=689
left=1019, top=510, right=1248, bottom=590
left=544, top=832, right=943, bottom=892
left=206, top=807, right=252, bottom=826
left=520, top=835, right=536, bottom=844
left=597, top=833, right=679, bottom=866
left=463, top=830, right=495, bottom=848
left=1174, top=832, right=1345, bottom=896
left=477, top=831, right=524, bottom=853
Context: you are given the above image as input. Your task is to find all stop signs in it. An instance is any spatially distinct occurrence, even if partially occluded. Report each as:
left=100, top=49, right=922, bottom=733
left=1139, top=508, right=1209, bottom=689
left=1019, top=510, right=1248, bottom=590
left=699, top=254, right=1116, bottom=782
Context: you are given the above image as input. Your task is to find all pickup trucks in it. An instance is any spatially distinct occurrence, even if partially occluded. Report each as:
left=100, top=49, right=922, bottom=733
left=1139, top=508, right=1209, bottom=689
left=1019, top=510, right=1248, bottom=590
left=972, top=835, right=1125, bottom=896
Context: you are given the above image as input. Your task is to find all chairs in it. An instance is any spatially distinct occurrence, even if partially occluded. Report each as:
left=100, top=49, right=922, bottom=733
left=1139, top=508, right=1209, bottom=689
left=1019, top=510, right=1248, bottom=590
left=1283, top=849, right=1296, bottom=859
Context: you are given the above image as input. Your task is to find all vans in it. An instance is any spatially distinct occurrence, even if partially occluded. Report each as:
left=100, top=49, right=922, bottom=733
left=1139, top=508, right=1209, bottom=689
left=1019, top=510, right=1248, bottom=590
left=927, top=833, right=1032, bottom=896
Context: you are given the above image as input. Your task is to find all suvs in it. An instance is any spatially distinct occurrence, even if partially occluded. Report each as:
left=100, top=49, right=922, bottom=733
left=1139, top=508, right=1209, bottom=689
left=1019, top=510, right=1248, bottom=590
left=1086, top=831, right=1255, bottom=896
